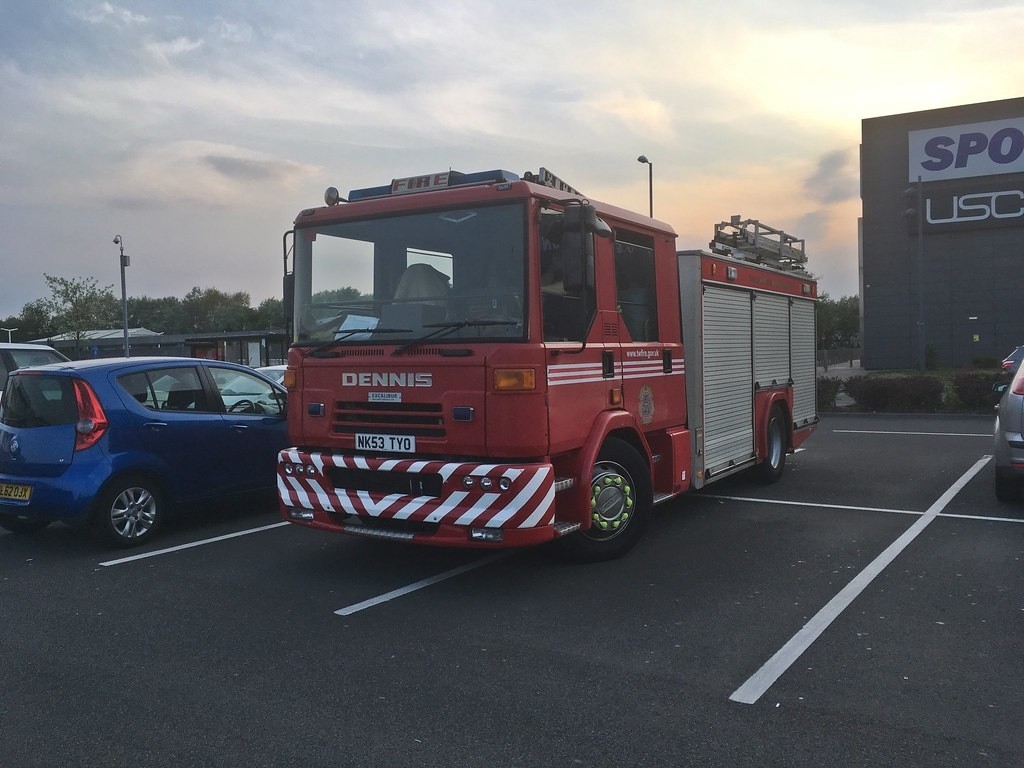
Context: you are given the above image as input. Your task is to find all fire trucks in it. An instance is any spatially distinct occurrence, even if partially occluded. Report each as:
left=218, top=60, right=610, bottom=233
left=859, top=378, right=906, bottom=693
left=273, top=167, right=820, bottom=557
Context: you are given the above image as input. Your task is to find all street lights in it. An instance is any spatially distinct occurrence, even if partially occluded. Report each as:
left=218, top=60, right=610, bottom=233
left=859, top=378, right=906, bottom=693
left=1, top=328, right=18, bottom=343
left=638, top=156, right=653, bottom=217
left=113, top=234, right=129, bottom=358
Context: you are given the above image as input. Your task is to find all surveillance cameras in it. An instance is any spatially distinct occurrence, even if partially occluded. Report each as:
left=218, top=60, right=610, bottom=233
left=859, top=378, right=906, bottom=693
left=113, top=238, right=120, bottom=244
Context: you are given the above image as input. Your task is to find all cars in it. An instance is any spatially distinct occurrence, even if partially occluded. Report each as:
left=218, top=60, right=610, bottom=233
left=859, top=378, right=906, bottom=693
left=191, top=364, right=286, bottom=413
left=994, top=356, right=1024, bottom=498
left=142, top=366, right=250, bottom=412
left=991, top=344, right=1024, bottom=397
left=0, top=342, right=72, bottom=415
left=0, top=357, right=290, bottom=549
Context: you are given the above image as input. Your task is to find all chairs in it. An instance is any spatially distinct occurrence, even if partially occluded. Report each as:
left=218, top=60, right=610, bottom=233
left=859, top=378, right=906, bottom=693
left=397, top=264, right=453, bottom=313
left=165, top=384, right=197, bottom=411
left=129, top=385, right=156, bottom=410
